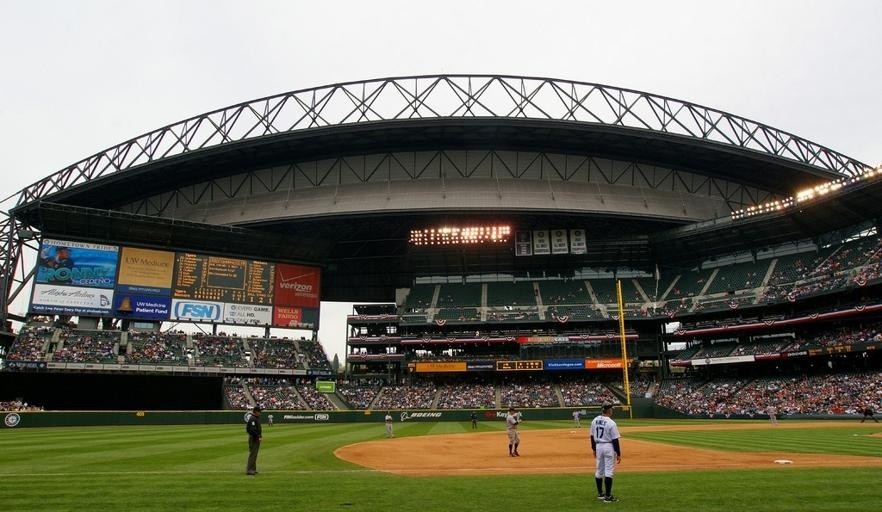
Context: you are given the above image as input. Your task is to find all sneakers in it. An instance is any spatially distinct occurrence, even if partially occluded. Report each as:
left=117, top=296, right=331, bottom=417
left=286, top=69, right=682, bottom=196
left=509, top=450, right=520, bottom=458
left=597, top=494, right=620, bottom=503
left=247, top=469, right=258, bottom=475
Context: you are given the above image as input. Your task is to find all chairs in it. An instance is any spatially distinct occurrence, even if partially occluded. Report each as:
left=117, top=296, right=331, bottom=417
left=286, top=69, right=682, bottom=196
left=0, top=313, right=395, bottom=411
left=395, top=231, right=882, bottom=415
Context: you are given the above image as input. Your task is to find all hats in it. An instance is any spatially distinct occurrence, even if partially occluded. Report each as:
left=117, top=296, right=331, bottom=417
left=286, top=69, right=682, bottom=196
left=601, top=400, right=613, bottom=408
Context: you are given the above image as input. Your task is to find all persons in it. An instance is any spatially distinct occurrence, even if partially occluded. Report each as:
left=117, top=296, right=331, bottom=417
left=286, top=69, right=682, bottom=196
left=471, top=410, right=479, bottom=432
left=337, top=286, right=661, bottom=411
left=1, top=310, right=339, bottom=411
left=572, top=410, right=581, bottom=428
left=591, top=401, right=622, bottom=503
left=651, top=243, right=882, bottom=414
left=768, top=402, right=781, bottom=427
left=384, top=412, right=394, bottom=438
left=506, top=407, right=521, bottom=457
left=268, top=415, right=274, bottom=427
left=861, top=407, right=878, bottom=422
left=246, top=406, right=262, bottom=475
left=41, top=246, right=74, bottom=270
